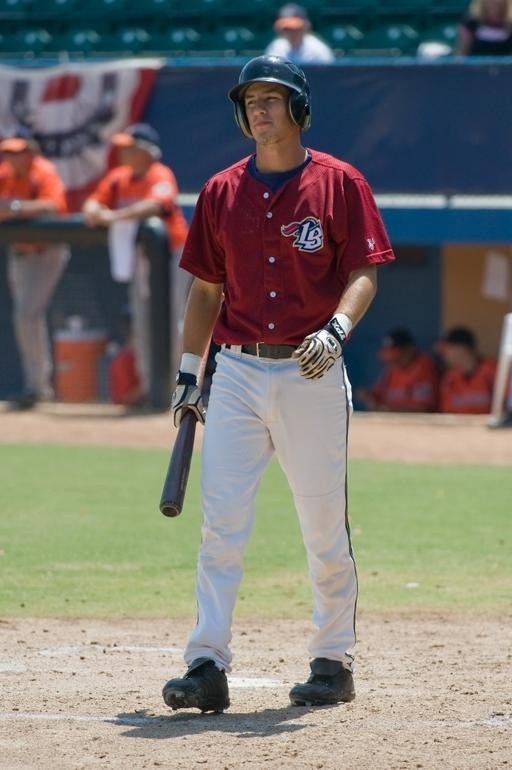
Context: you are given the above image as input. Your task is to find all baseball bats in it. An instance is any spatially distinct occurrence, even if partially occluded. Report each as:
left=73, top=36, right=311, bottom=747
left=159, top=408, right=195, bottom=518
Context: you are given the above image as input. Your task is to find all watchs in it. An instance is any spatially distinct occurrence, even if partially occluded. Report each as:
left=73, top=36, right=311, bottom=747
left=8, top=199, right=22, bottom=221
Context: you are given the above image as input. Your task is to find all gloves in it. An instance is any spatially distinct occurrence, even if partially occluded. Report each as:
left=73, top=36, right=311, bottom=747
left=172, top=371, right=206, bottom=427
left=293, top=318, right=346, bottom=379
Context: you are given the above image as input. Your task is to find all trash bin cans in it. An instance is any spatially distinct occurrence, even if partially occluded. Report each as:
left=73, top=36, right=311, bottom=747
left=54, top=330, right=109, bottom=402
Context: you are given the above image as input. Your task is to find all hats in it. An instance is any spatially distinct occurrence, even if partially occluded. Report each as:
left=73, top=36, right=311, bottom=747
left=379, top=328, right=474, bottom=362
left=112, top=122, right=161, bottom=148
left=0, top=124, right=32, bottom=153
left=274, top=5, right=306, bottom=30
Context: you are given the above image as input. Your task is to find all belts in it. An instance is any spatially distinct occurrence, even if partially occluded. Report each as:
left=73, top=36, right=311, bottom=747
left=225, top=344, right=297, bottom=359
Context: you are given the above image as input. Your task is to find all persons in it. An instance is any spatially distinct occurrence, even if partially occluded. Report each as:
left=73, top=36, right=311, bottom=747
left=263, top=4, right=334, bottom=62
left=456, top=0, right=512, bottom=55
left=353, top=324, right=441, bottom=413
left=435, top=328, right=510, bottom=415
left=79, top=123, right=192, bottom=411
left=1, top=124, right=75, bottom=409
left=161, top=56, right=397, bottom=713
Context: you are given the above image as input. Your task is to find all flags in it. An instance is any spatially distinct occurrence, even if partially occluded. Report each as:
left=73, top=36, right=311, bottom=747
left=0, top=59, right=163, bottom=212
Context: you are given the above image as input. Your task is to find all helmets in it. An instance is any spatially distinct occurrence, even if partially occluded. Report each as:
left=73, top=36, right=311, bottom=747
left=228, top=56, right=311, bottom=138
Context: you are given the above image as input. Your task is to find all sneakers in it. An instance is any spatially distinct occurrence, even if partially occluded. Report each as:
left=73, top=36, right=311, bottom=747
left=288, top=658, right=355, bottom=705
left=162, top=656, right=229, bottom=712
